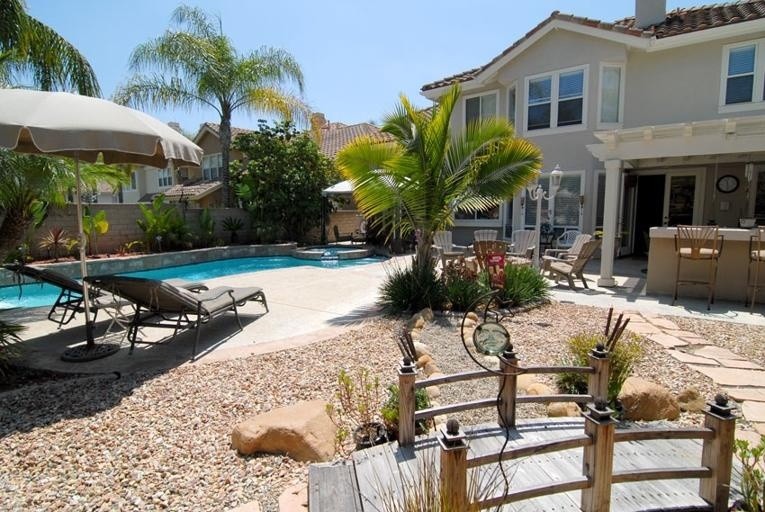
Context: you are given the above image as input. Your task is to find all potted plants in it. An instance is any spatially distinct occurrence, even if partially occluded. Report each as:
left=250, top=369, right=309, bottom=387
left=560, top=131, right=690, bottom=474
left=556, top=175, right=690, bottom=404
left=595, top=228, right=629, bottom=261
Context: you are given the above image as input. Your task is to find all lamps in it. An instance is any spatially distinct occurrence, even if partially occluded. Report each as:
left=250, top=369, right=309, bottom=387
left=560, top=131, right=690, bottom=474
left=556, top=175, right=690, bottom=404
left=578, top=196, right=583, bottom=215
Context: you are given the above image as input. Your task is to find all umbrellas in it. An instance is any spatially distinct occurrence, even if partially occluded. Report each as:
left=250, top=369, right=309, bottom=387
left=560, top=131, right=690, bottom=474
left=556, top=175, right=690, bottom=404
left=322, top=165, right=419, bottom=244
left=0, top=86, right=205, bottom=345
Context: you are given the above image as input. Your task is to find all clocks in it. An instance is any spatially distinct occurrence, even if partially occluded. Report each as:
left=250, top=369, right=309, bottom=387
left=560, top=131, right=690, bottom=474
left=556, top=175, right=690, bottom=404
left=716, top=175, right=739, bottom=193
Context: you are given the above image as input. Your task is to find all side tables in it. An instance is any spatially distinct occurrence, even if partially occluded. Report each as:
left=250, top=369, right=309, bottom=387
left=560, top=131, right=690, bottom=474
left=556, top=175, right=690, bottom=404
left=91, top=294, right=148, bottom=347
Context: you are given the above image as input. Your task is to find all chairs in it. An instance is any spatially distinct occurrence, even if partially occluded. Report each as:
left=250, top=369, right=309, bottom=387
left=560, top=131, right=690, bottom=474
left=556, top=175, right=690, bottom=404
left=672, top=224, right=724, bottom=311
left=744, top=225, right=765, bottom=312
left=415, top=228, right=600, bottom=290
left=1, top=264, right=210, bottom=325
left=333, top=225, right=352, bottom=245
left=83, top=274, right=269, bottom=364
